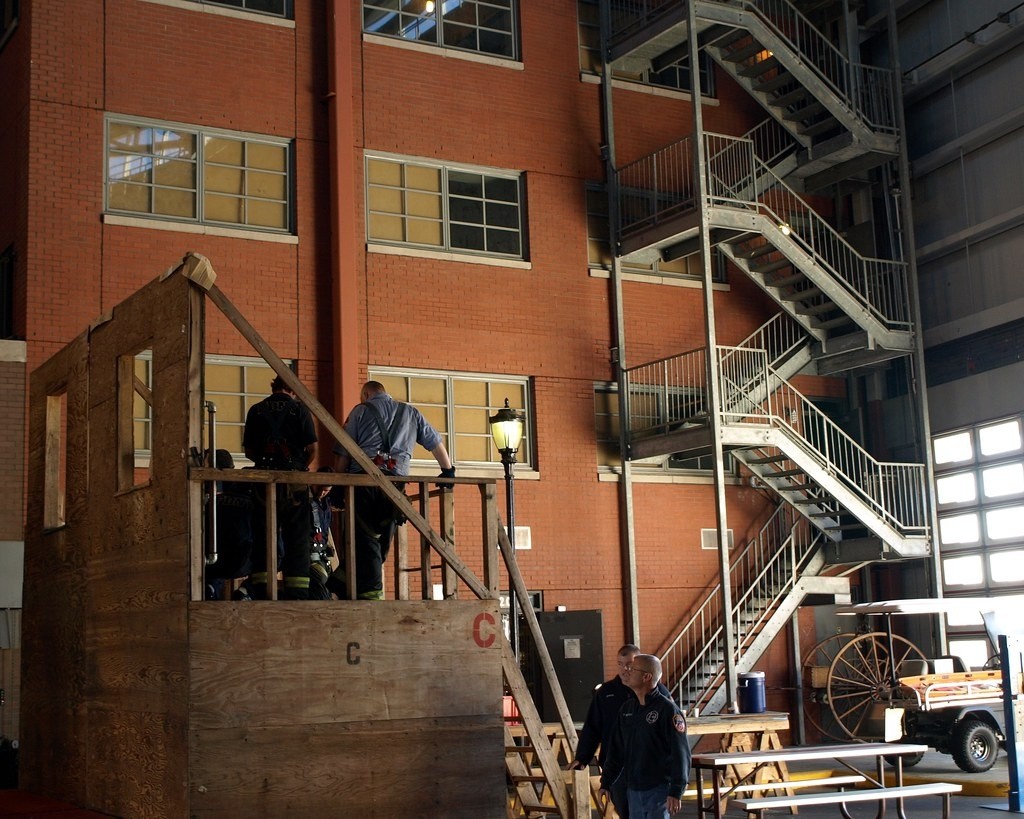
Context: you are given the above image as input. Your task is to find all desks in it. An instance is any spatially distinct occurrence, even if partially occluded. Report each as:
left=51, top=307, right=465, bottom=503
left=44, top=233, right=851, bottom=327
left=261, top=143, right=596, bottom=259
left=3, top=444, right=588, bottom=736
left=500, top=711, right=799, bottom=819
left=691, top=743, right=929, bottom=819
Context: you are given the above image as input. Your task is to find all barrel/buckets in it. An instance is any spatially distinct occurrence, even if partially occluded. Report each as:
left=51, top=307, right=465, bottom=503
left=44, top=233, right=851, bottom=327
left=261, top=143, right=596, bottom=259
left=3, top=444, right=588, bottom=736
left=737, top=672, right=766, bottom=712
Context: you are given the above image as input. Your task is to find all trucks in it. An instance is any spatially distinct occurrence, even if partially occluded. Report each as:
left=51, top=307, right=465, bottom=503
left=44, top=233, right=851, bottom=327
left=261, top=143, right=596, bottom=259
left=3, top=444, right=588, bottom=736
left=874, top=652, right=1024, bottom=774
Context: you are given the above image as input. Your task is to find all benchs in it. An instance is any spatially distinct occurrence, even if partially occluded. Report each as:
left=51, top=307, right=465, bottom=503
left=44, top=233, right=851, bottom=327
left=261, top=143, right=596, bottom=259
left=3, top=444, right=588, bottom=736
left=680, top=776, right=867, bottom=819
left=728, top=782, right=963, bottom=819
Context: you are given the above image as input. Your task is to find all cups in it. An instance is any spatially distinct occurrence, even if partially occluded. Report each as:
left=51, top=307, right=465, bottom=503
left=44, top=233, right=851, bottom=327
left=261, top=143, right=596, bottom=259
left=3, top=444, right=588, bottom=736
left=692, top=708, right=700, bottom=718
left=681, top=710, right=686, bottom=718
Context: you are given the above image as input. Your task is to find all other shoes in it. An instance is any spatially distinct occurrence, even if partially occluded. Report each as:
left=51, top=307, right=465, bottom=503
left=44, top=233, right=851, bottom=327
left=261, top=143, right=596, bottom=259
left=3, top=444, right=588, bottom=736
left=232, top=589, right=250, bottom=601
left=205, top=585, right=215, bottom=600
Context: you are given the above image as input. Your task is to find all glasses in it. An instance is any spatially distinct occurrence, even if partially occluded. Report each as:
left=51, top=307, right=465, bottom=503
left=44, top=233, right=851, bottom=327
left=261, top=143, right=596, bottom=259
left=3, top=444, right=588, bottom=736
left=623, top=665, right=646, bottom=675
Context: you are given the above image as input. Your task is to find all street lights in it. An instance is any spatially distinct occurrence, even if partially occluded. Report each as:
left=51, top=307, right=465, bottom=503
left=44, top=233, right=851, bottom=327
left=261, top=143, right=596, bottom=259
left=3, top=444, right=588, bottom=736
left=487, top=396, right=525, bottom=686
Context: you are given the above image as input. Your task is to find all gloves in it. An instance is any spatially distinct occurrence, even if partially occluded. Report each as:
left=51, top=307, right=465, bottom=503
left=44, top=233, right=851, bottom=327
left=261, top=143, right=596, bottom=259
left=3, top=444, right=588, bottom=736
left=435, top=467, right=456, bottom=489
left=329, top=490, right=345, bottom=510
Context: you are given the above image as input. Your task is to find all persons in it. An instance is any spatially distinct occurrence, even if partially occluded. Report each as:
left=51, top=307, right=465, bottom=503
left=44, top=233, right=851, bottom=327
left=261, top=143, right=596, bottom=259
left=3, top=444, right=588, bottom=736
left=241, top=375, right=318, bottom=601
left=326, top=381, right=455, bottom=600
left=204, top=448, right=336, bottom=601
left=566, top=644, right=673, bottom=819
left=598, top=654, right=692, bottom=819
